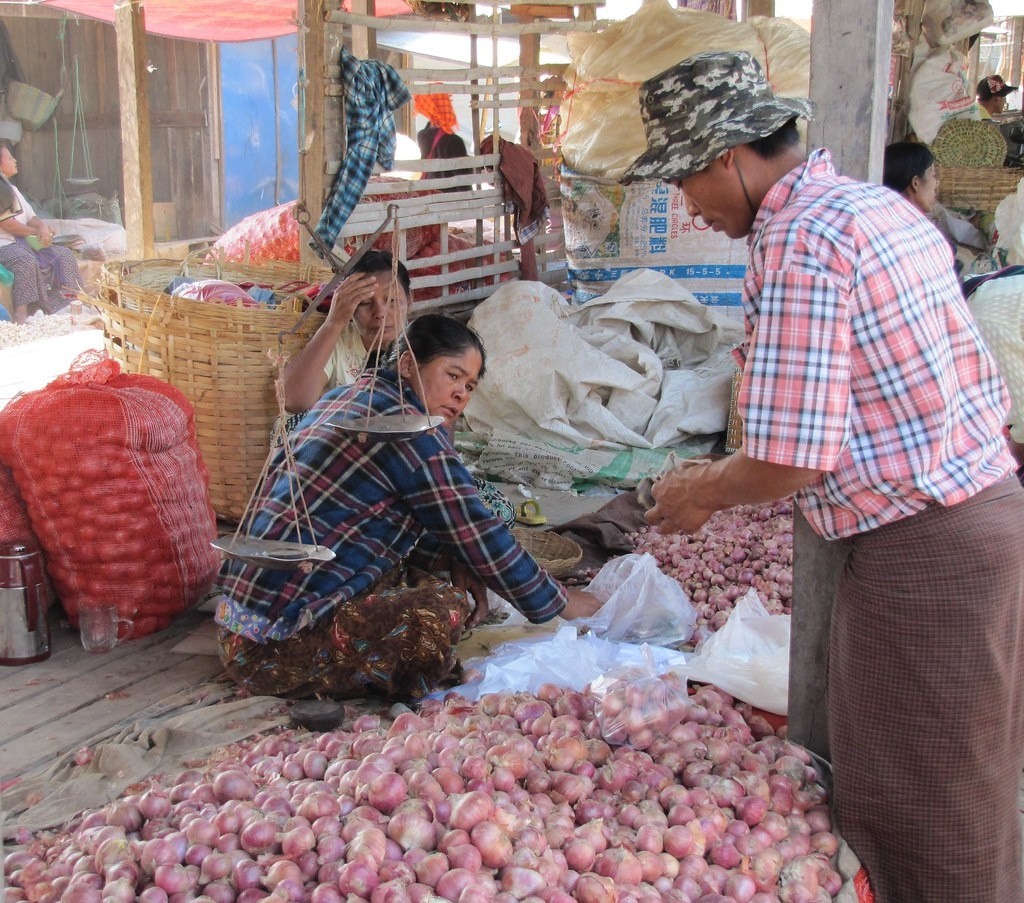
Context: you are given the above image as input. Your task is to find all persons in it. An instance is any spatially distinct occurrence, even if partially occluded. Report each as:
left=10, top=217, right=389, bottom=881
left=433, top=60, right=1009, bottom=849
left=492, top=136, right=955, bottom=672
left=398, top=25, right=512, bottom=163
left=211, top=313, right=605, bottom=708
left=0, top=144, right=83, bottom=325
left=619, top=50, right=1024, bottom=901
left=417, top=121, right=473, bottom=194
left=974, top=73, right=1018, bottom=123
left=268, top=250, right=515, bottom=526
left=883, top=142, right=964, bottom=286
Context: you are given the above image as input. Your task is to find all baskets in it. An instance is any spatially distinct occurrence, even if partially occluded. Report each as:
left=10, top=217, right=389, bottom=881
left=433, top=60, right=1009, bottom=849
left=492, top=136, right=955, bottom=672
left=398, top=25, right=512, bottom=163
left=930, top=166, right=1024, bottom=212
left=507, top=528, right=583, bottom=579
left=63, top=258, right=340, bottom=524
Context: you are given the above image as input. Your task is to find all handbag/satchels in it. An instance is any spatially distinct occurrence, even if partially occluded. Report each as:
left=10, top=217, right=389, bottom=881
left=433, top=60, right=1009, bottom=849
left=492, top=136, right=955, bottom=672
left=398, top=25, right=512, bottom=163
left=6, top=77, right=64, bottom=131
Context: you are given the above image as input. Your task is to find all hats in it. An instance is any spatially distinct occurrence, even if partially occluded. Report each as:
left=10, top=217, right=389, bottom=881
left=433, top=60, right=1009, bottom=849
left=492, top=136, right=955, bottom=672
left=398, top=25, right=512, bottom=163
left=617, top=50, right=818, bottom=186
left=976, top=74, right=1018, bottom=97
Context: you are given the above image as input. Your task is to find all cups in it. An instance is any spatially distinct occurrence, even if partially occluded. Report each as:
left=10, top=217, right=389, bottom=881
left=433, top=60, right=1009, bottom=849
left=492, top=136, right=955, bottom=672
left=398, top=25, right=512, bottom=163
left=79, top=601, right=134, bottom=653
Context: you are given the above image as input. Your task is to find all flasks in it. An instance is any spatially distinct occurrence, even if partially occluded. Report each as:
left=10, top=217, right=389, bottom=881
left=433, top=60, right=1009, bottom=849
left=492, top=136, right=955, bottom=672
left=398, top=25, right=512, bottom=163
left=0, top=542, right=52, bottom=666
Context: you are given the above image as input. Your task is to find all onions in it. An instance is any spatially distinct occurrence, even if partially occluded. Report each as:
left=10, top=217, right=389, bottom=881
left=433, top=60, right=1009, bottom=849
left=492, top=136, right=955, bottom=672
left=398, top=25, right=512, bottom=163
left=0, top=377, right=221, bottom=649
left=3, top=681, right=845, bottom=903
left=199, top=176, right=514, bottom=306
left=563, top=495, right=794, bottom=648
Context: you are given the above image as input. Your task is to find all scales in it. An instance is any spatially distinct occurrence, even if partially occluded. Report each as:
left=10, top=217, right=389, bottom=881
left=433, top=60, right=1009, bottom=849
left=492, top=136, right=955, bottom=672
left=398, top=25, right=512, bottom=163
left=44, top=11, right=100, bottom=245
left=208, top=203, right=447, bottom=569
